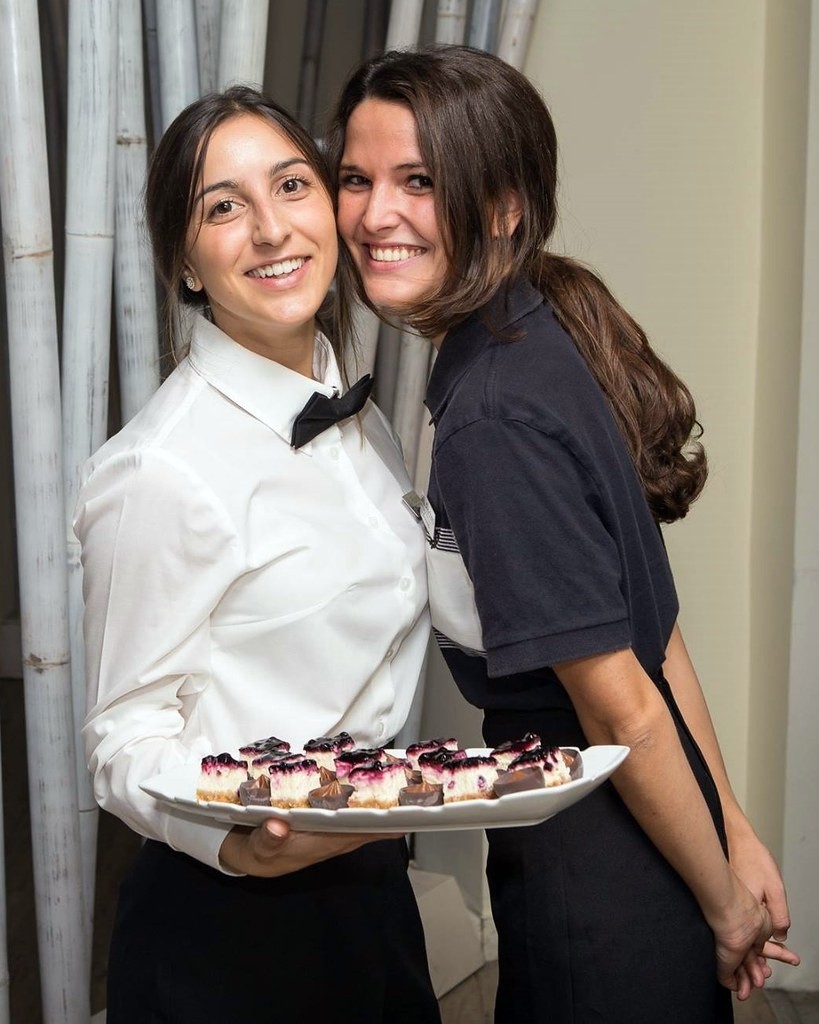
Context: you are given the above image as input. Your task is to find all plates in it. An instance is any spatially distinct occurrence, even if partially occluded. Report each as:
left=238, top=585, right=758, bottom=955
left=136, top=745, right=630, bottom=835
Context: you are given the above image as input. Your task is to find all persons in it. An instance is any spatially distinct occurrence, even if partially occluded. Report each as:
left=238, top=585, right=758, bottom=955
left=73, top=87, right=449, bottom=1024
left=321, top=42, right=799, bottom=1024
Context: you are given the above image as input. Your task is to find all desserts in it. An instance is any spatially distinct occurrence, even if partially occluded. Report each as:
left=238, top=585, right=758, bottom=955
left=193, top=731, right=583, bottom=811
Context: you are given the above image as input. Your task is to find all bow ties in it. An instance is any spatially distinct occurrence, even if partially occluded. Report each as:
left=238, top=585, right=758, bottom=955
left=290, top=373, right=375, bottom=449
